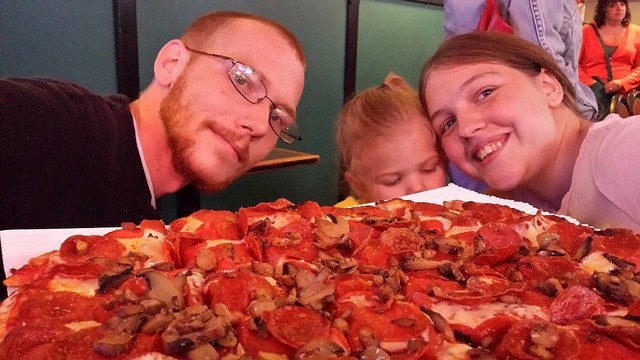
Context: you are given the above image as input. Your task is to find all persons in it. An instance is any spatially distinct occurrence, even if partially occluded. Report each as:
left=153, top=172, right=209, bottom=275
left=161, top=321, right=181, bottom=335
left=331, top=73, right=447, bottom=210
left=1, top=11, right=306, bottom=302
left=418, top=31, right=640, bottom=235
left=442, top=0, right=599, bottom=122
left=577, top=1, right=640, bottom=118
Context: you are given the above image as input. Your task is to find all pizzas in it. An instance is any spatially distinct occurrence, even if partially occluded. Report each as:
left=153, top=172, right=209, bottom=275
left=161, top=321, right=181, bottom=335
left=0, top=197, right=640, bottom=360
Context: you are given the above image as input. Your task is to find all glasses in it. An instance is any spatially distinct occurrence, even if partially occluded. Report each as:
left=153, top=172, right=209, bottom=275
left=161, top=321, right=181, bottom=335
left=182, top=44, right=302, bottom=145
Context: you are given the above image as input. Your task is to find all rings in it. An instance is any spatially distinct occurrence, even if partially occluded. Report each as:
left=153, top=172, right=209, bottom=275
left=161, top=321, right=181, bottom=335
left=610, top=87, right=613, bottom=91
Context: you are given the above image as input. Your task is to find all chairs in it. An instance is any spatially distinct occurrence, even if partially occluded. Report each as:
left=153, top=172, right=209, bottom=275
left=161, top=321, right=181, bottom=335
left=609, top=89, right=640, bottom=117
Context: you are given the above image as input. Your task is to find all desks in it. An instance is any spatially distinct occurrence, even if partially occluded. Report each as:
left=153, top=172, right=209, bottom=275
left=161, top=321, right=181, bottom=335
left=245, top=146, right=320, bottom=174
